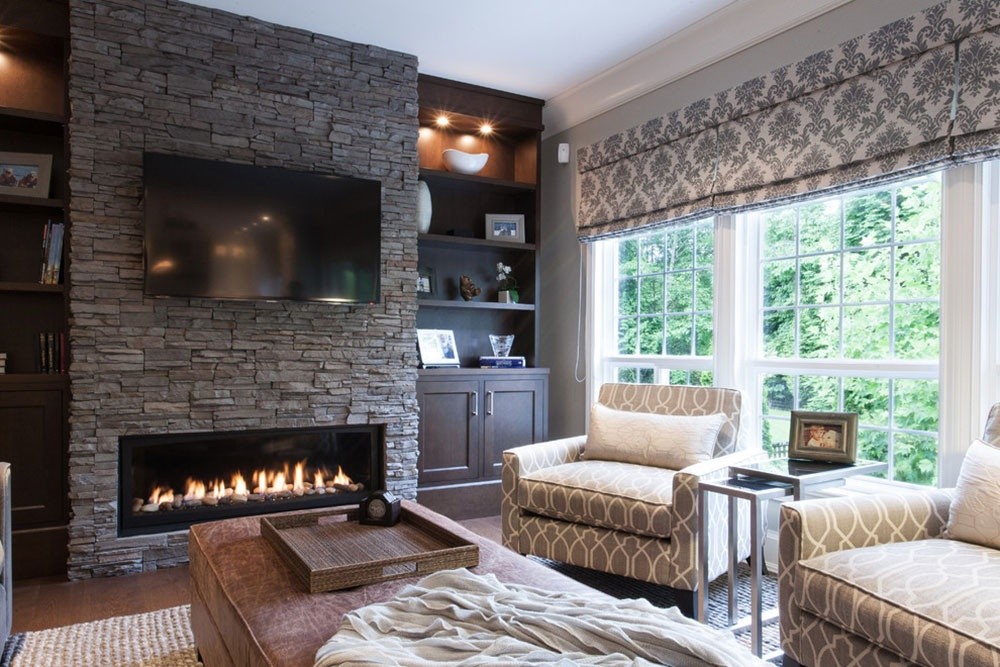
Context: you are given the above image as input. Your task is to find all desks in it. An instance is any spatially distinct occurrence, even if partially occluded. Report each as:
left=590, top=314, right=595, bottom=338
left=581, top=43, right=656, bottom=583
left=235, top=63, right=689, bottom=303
left=695, top=457, right=891, bottom=663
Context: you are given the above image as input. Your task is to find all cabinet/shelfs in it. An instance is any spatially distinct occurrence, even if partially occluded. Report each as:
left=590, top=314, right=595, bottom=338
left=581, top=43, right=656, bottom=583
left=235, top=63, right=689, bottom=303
left=0, top=0, right=73, bottom=575
left=416, top=103, right=548, bottom=484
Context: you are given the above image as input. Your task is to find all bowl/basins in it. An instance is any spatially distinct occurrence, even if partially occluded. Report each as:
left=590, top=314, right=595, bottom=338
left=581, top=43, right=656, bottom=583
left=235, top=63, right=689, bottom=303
left=417, top=179, right=432, bottom=235
left=442, top=149, right=489, bottom=175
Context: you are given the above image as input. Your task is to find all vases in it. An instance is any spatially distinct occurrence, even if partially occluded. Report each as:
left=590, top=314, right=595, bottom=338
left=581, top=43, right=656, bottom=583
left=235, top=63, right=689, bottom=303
left=499, top=291, right=516, bottom=304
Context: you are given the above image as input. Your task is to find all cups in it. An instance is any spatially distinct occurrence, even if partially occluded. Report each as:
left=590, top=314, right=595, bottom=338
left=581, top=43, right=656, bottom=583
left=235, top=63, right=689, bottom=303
left=489, top=334, right=515, bottom=357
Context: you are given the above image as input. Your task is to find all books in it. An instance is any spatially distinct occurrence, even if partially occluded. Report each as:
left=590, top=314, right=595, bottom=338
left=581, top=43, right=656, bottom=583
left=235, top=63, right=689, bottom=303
left=0, top=353, right=7, bottom=373
left=33, top=331, right=65, bottom=374
left=37, top=220, right=64, bottom=284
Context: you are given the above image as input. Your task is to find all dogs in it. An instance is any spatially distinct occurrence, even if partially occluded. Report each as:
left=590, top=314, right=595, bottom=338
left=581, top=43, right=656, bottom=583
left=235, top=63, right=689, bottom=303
left=821, top=430, right=841, bottom=448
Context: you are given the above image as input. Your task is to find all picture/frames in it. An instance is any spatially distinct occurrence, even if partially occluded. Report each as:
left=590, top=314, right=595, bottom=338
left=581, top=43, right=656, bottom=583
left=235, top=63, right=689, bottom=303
left=0, top=152, right=54, bottom=199
left=416, top=267, right=437, bottom=300
left=788, top=410, right=859, bottom=463
left=485, top=213, right=525, bottom=243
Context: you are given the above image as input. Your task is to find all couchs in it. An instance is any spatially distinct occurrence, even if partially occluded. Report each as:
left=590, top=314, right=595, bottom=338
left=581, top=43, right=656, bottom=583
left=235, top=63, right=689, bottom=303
left=778, top=401, right=1000, bottom=667
left=500, top=382, right=768, bottom=622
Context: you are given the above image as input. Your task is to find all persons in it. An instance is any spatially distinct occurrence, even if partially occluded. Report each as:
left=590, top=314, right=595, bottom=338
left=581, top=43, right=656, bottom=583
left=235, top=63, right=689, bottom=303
left=807, top=425, right=825, bottom=448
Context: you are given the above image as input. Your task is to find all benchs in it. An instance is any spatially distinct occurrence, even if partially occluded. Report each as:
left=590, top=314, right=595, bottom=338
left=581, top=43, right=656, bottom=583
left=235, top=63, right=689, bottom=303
left=188, top=496, right=622, bottom=667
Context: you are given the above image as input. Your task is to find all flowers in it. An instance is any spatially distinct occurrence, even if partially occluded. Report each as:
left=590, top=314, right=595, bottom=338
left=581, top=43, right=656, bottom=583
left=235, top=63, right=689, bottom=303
left=496, top=261, right=520, bottom=303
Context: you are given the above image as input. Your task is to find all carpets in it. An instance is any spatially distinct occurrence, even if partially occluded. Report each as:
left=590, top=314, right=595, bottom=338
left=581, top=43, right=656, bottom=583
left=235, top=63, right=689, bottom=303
left=3, top=553, right=805, bottom=667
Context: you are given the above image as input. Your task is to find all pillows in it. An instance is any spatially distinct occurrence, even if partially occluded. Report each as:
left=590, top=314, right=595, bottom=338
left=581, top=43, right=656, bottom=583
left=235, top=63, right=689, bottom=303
left=580, top=400, right=726, bottom=470
left=942, top=439, right=1000, bottom=551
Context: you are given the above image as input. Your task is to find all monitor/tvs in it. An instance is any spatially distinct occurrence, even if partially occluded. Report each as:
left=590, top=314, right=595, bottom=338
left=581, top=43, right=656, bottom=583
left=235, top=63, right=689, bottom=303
left=143, top=150, right=382, bottom=305
